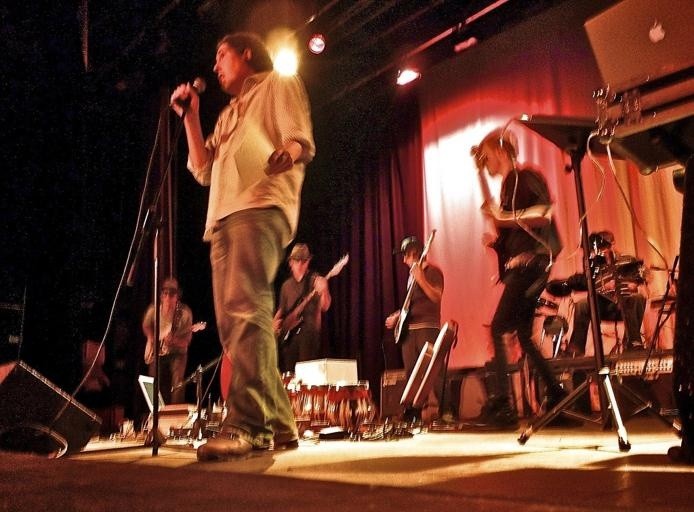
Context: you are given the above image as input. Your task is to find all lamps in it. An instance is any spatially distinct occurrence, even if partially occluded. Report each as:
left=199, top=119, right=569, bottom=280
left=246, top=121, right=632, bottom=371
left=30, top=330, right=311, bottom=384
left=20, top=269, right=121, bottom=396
left=307, top=15, right=329, bottom=54
left=394, top=50, right=424, bottom=89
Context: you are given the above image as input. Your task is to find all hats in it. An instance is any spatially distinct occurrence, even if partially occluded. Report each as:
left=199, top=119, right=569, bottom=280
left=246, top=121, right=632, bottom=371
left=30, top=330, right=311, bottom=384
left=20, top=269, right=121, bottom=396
left=286, top=243, right=314, bottom=264
left=158, top=278, right=184, bottom=298
left=391, top=235, right=426, bottom=256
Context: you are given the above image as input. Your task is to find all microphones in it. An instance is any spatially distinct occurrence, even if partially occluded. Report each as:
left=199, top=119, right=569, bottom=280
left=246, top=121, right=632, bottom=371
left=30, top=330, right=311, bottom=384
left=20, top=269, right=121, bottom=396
left=171, top=75, right=206, bottom=106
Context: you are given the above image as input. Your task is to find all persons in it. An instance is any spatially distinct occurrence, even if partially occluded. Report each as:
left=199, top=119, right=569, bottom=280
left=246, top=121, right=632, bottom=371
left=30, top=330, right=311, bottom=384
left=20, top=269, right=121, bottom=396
left=274, top=242, right=333, bottom=371
left=468, top=123, right=577, bottom=428
left=168, top=27, right=316, bottom=458
left=143, top=277, right=194, bottom=405
left=384, top=236, right=458, bottom=423
left=565, top=227, right=650, bottom=355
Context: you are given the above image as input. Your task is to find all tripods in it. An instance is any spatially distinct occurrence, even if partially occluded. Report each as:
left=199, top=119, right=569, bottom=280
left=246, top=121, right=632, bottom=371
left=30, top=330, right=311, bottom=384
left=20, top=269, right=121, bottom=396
left=518, top=156, right=681, bottom=452
left=165, top=346, right=221, bottom=448
left=71, top=110, right=204, bottom=456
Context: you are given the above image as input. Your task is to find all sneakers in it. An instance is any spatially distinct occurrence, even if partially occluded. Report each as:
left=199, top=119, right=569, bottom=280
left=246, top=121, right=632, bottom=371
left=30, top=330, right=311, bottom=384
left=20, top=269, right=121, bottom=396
left=197, top=423, right=301, bottom=466
left=533, top=393, right=586, bottom=430
left=460, top=401, right=517, bottom=430
left=392, top=404, right=426, bottom=426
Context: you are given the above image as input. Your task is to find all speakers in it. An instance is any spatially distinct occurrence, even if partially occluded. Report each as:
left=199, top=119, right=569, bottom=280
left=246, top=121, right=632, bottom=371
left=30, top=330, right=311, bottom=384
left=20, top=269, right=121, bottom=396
left=0, top=360, right=102, bottom=457
left=377, top=370, right=423, bottom=422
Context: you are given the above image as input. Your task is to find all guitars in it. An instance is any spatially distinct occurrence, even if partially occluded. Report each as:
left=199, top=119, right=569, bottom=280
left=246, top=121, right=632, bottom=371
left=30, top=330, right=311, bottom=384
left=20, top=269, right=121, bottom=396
left=278, top=254, right=349, bottom=347
left=393, top=229, right=435, bottom=345
left=144, top=321, right=209, bottom=365
